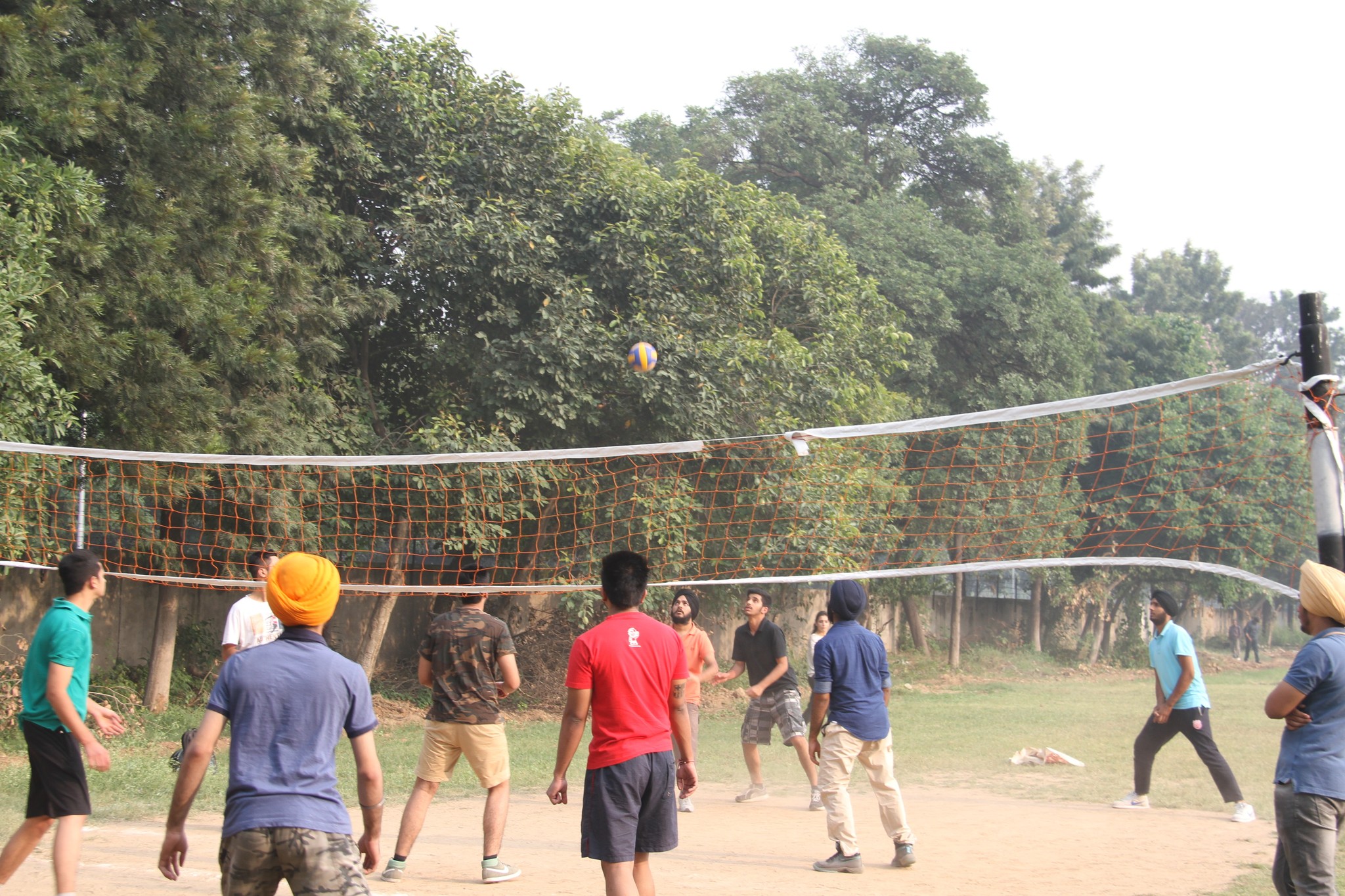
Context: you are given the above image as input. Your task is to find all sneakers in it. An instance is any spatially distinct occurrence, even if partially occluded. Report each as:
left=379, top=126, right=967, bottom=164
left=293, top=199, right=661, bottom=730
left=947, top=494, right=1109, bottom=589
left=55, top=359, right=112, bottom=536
left=1112, top=791, right=1150, bottom=809
left=380, top=858, right=407, bottom=882
left=678, top=794, right=694, bottom=812
left=1231, top=800, right=1255, bottom=822
left=809, top=788, right=825, bottom=811
left=891, top=839, right=916, bottom=867
left=812, top=841, right=864, bottom=873
left=736, top=783, right=768, bottom=802
left=481, top=857, right=521, bottom=883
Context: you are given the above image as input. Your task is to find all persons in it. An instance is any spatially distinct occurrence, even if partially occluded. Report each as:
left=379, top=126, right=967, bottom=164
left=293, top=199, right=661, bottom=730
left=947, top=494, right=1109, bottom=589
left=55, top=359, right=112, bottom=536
left=808, top=580, right=916, bottom=874
left=1227, top=615, right=1262, bottom=663
left=223, top=550, right=284, bottom=664
left=663, top=589, right=718, bottom=813
left=158, top=553, right=385, bottom=896
left=1262, top=559, right=1344, bottom=896
left=546, top=550, right=698, bottom=896
left=1, top=550, right=130, bottom=896
left=801, top=611, right=834, bottom=726
left=381, top=564, right=521, bottom=884
left=1114, top=589, right=1254, bottom=824
left=706, top=588, right=824, bottom=811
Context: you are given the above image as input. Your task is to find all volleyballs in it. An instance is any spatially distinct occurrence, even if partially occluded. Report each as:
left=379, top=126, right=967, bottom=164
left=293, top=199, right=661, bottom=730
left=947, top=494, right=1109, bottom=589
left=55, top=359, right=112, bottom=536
left=628, top=341, right=657, bottom=372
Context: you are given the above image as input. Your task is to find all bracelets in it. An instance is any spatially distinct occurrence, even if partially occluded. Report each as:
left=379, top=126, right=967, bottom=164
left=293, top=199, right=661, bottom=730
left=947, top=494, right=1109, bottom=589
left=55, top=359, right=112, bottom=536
left=676, top=759, right=695, bottom=769
left=359, top=797, right=384, bottom=808
